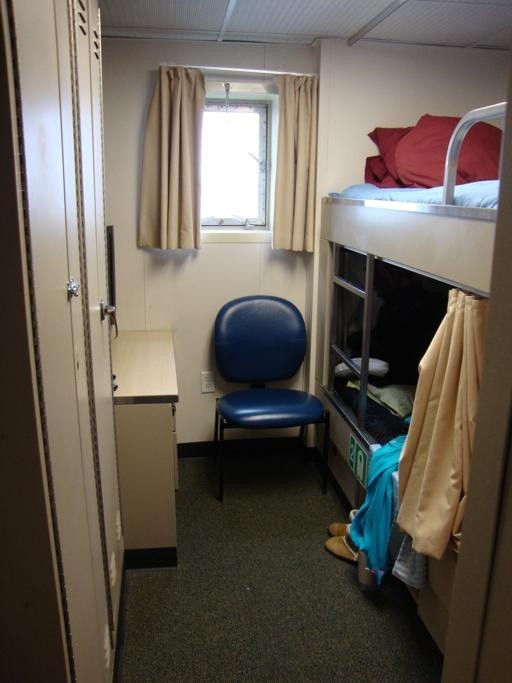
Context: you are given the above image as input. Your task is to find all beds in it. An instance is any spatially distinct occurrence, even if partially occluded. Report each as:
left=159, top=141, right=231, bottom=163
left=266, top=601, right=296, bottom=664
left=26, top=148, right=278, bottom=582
left=315, top=100, right=511, bottom=657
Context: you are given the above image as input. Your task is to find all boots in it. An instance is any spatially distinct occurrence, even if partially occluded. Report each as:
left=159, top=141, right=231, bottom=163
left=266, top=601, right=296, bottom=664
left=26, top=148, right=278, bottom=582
left=324, top=508, right=362, bottom=564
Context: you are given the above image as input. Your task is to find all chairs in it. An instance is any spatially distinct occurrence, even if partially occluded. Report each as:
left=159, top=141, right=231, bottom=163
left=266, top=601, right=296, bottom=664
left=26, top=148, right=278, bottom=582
left=213, top=295, right=331, bottom=505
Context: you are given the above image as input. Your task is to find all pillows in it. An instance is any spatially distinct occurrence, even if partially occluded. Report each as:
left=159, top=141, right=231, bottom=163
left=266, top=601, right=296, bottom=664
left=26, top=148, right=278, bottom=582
left=364, top=113, right=505, bottom=188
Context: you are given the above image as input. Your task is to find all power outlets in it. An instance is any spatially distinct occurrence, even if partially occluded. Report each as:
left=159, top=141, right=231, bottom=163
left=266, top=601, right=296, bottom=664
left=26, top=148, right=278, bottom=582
left=200, top=371, right=216, bottom=393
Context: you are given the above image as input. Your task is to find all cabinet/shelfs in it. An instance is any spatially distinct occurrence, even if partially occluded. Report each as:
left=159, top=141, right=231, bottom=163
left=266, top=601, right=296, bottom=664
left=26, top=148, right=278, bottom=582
left=111, top=326, right=181, bottom=573
